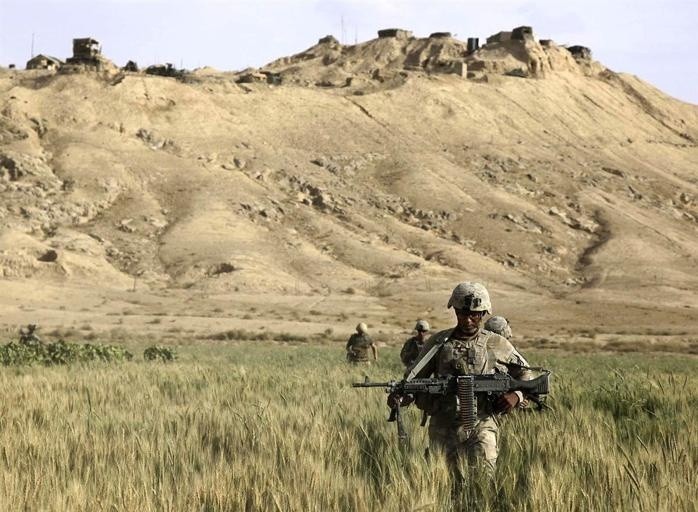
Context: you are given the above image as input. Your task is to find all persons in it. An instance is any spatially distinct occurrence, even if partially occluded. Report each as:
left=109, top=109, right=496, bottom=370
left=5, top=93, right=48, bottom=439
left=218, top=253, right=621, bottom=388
left=399, top=318, right=430, bottom=369
left=344, top=322, right=377, bottom=367
left=386, top=281, right=532, bottom=504
left=484, top=314, right=512, bottom=340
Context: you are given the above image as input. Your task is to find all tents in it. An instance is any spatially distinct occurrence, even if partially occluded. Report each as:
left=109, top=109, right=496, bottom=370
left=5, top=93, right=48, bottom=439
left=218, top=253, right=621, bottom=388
left=26, top=53, right=65, bottom=70
left=145, top=64, right=166, bottom=74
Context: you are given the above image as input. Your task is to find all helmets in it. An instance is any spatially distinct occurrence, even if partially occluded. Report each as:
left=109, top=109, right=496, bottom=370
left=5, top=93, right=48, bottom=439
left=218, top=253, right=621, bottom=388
left=484, top=316, right=512, bottom=338
left=356, top=322, right=366, bottom=333
left=415, top=320, right=428, bottom=331
left=448, top=283, right=492, bottom=314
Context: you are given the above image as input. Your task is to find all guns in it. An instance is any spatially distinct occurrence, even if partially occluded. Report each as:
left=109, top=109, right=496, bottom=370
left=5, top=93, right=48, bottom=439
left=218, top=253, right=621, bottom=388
left=353, top=374, right=549, bottom=431
